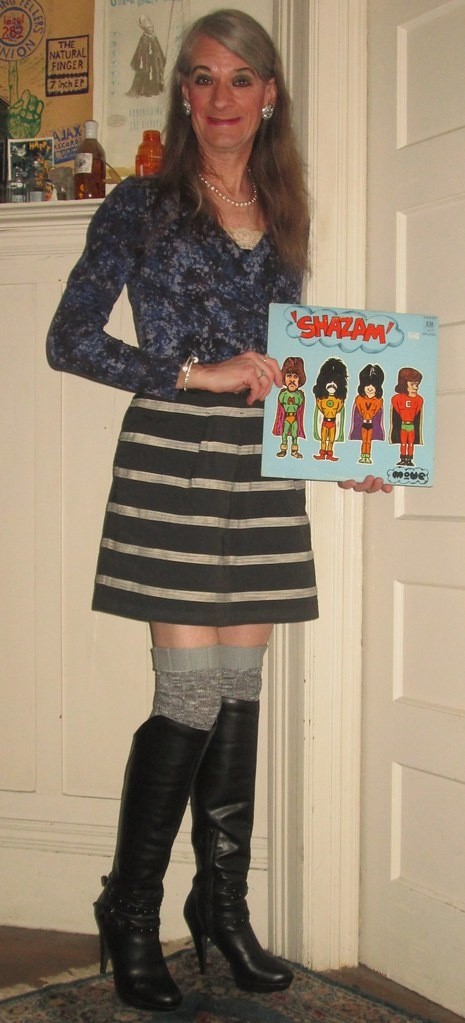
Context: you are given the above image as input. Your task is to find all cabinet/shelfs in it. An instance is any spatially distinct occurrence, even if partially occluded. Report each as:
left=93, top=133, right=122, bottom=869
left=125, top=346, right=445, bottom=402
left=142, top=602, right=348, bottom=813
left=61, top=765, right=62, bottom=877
left=0, top=199, right=270, bottom=944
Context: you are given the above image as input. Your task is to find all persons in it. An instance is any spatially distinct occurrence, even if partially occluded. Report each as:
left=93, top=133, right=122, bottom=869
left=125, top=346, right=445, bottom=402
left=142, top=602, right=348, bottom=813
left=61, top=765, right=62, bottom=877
left=46, top=9, right=394, bottom=1010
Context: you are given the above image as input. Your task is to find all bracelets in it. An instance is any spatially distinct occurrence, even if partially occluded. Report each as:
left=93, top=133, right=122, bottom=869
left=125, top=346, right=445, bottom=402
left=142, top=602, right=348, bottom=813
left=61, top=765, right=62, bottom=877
left=182, top=355, right=199, bottom=391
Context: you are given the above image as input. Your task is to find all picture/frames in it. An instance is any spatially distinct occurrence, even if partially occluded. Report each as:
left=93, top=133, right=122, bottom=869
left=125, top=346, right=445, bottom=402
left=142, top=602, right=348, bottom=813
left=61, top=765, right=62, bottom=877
left=93, top=0, right=274, bottom=197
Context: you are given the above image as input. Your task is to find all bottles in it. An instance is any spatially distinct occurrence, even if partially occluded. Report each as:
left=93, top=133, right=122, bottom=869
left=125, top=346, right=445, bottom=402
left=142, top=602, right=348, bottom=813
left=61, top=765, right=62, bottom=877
left=6, top=160, right=46, bottom=203
left=136, top=130, right=164, bottom=177
left=75, top=120, right=105, bottom=200
left=53, top=125, right=84, bottom=163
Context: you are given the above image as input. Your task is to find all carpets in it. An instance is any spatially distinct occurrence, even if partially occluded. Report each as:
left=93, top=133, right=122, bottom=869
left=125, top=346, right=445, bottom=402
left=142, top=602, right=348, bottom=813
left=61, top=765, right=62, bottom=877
left=0, top=935, right=441, bottom=1023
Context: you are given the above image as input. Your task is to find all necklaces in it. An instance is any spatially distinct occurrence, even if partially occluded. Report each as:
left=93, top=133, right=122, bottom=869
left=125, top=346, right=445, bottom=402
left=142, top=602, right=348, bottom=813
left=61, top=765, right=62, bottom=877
left=198, top=166, right=257, bottom=207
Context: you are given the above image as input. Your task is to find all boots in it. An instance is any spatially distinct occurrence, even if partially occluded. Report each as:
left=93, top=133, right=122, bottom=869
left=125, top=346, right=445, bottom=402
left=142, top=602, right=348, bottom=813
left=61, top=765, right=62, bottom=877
left=93, top=714, right=218, bottom=1012
left=182, top=694, right=293, bottom=993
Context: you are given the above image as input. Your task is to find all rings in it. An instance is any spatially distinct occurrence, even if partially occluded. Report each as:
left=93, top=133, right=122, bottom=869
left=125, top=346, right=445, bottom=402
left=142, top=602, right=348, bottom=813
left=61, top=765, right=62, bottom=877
left=263, top=353, right=269, bottom=363
left=258, top=370, right=267, bottom=379
